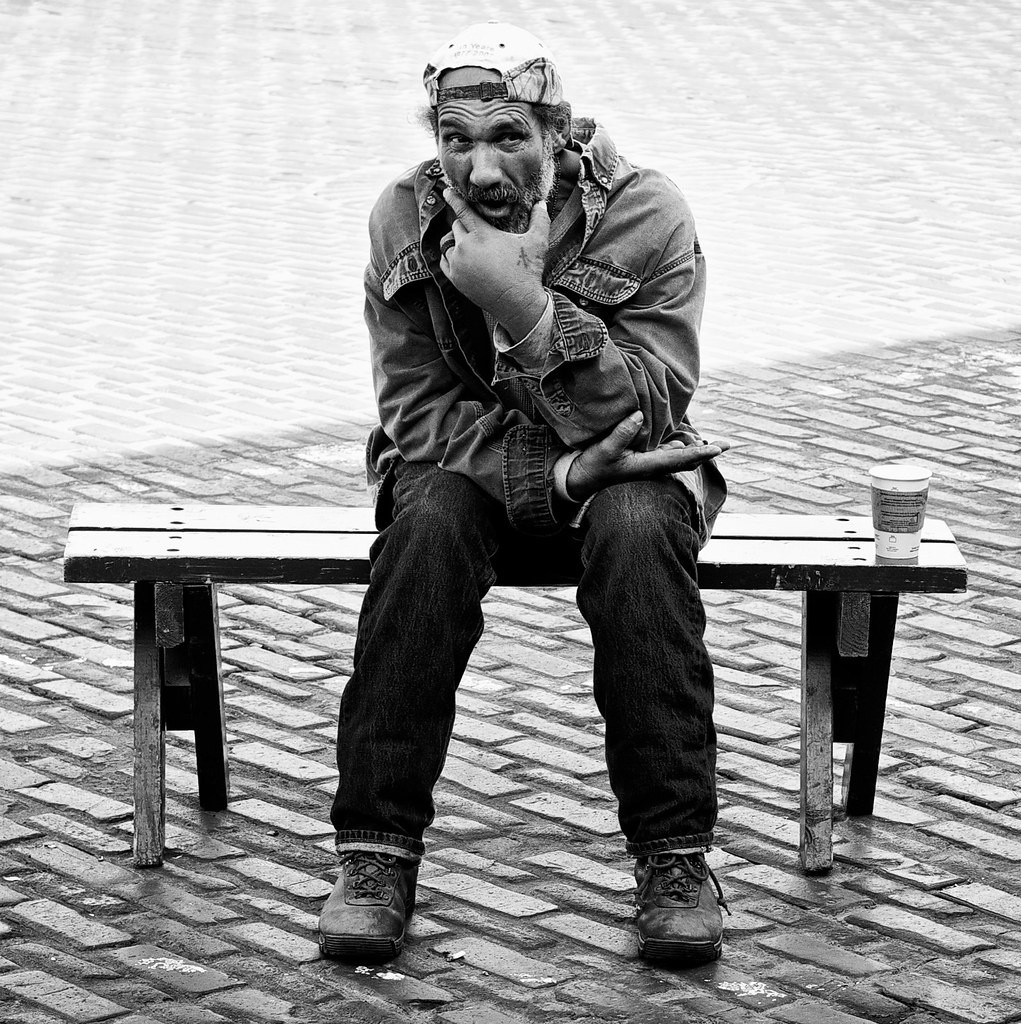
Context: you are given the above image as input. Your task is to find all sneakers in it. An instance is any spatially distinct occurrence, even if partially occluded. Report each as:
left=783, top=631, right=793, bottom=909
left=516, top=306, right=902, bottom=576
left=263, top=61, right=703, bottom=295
left=316, top=851, right=422, bottom=954
left=629, top=852, right=732, bottom=960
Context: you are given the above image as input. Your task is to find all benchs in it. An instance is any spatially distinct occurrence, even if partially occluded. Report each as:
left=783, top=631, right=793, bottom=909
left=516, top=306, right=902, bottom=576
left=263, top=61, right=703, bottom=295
left=62, top=500, right=972, bottom=879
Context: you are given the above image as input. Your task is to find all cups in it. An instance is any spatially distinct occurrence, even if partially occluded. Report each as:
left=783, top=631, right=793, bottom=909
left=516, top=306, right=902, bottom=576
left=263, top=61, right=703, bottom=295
left=869, top=466, right=932, bottom=559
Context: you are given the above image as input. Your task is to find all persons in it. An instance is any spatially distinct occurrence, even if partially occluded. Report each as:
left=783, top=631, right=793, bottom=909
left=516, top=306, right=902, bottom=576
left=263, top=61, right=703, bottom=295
left=311, top=22, right=731, bottom=959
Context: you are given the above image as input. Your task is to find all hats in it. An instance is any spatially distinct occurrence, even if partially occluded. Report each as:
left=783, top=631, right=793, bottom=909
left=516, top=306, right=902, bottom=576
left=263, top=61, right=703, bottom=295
left=422, top=19, right=564, bottom=106
